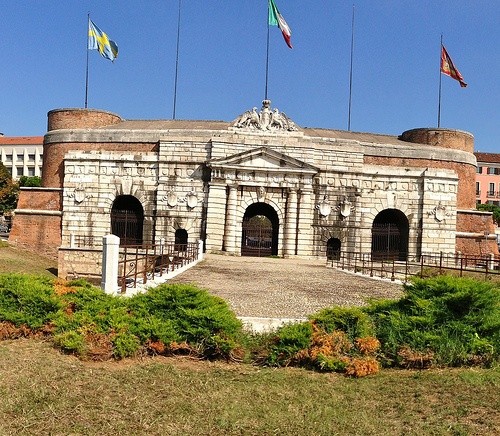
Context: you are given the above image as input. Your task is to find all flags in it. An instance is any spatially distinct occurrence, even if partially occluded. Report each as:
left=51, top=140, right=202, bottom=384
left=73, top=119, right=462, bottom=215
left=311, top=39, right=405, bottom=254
left=440, top=43, right=466, bottom=87
left=268, top=0, right=292, bottom=49
left=88, top=20, right=119, bottom=63
